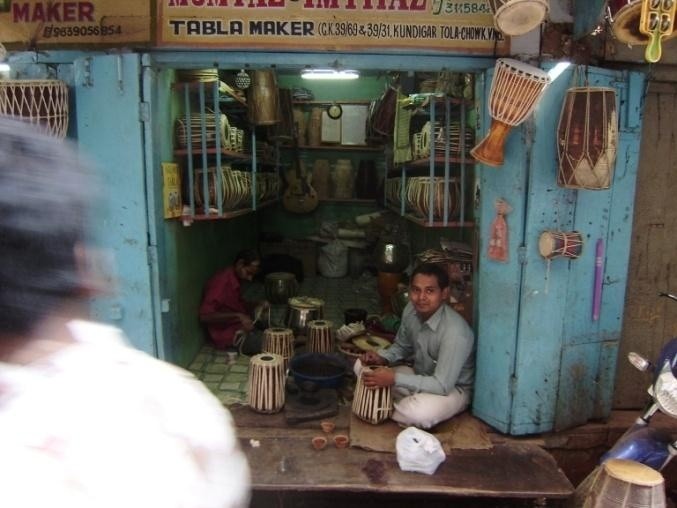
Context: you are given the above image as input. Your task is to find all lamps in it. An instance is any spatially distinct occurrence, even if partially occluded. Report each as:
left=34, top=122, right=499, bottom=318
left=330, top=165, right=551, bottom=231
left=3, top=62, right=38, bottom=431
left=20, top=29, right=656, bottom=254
left=300, top=69, right=361, bottom=79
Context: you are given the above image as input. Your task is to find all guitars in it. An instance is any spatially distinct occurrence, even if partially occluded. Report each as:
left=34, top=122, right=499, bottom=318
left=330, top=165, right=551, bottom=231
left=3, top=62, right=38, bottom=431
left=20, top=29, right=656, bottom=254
left=284, top=130, right=322, bottom=213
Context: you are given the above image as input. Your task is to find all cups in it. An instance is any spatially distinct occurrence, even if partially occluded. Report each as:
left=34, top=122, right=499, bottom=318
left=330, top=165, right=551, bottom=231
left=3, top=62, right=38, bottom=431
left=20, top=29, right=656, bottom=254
left=333, top=434, right=350, bottom=449
left=311, top=436, right=328, bottom=450
left=321, top=422, right=336, bottom=433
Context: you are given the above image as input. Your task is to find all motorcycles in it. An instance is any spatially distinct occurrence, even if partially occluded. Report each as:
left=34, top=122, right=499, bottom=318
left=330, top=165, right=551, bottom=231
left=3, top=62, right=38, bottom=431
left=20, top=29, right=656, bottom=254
left=569, top=289, right=677, bottom=506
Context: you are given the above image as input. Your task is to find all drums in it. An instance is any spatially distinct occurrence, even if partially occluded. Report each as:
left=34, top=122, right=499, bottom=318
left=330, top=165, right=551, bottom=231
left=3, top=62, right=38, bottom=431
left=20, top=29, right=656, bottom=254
left=555, top=86, right=617, bottom=190
left=189, top=165, right=283, bottom=206
left=489, top=0, right=549, bottom=37
left=351, top=365, right=390, bottom=424
left=386, top=174, right=460, bottom=220
left=248, top=327, right=294, bottom=416
left=0, top=80, right=70, bottom=140
left=472, top=59, right=550, bottom=166
left=307, top=321, right=336, bottom=356
left=412, top=119, right=475, bottom=158
left=539, top=229, right=582, bottom=259
left=606, top=1, right=677, bottom=45
left=266, top=272, right=298, bottom=304
left=178, top=113, right=266, bottom=156
left=372, top=88, right=403, bottom=135
left=572, top=460, right=667, bottom=508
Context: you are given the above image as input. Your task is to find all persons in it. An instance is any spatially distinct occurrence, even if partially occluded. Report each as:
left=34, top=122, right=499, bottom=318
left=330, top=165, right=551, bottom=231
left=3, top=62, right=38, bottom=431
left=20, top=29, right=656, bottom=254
left=196, top=249, right=272, bottom=356
left=0, top=114, right=252, bottom=508
left=357, top=265, right=476, bottom=429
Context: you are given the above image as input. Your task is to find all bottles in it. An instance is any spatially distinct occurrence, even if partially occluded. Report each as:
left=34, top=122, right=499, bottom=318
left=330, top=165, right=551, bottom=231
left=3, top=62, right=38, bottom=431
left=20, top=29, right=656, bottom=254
left=344, top=308, right=368, bottom=326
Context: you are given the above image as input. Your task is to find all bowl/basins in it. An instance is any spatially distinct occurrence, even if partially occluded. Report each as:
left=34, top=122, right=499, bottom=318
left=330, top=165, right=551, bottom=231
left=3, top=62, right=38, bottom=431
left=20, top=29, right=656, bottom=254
left=287, top=352, right=350, bottom=393
